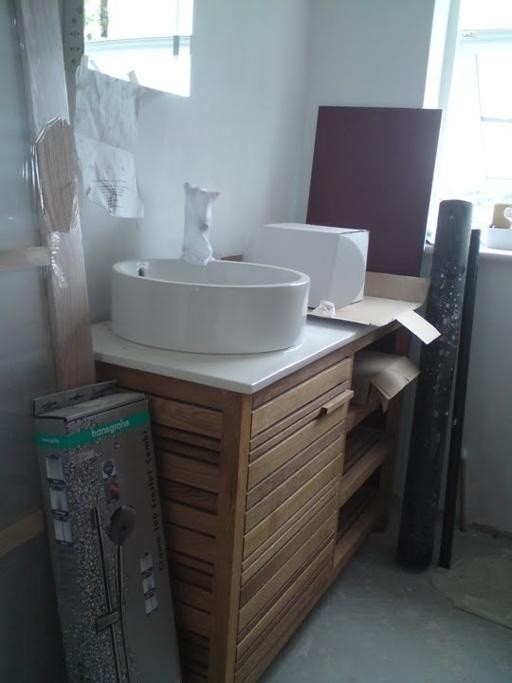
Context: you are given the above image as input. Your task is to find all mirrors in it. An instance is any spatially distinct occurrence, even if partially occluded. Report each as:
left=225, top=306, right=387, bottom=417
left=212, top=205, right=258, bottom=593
left=61, top=1, right=191, bottom=105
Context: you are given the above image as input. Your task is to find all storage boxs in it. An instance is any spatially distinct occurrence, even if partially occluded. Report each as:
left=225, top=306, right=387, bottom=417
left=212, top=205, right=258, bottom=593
left=261, top=221, right=370, bottom=308
left=31, top=378, right=182, bottom=682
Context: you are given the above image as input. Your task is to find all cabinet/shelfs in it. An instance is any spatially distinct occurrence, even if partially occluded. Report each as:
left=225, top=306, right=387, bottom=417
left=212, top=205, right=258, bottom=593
left=93, top=321, right=424, bottom=683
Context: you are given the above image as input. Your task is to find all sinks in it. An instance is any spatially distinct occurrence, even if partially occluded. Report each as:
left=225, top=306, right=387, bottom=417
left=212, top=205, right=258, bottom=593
left=110, top=258, right=310, bottom=353
